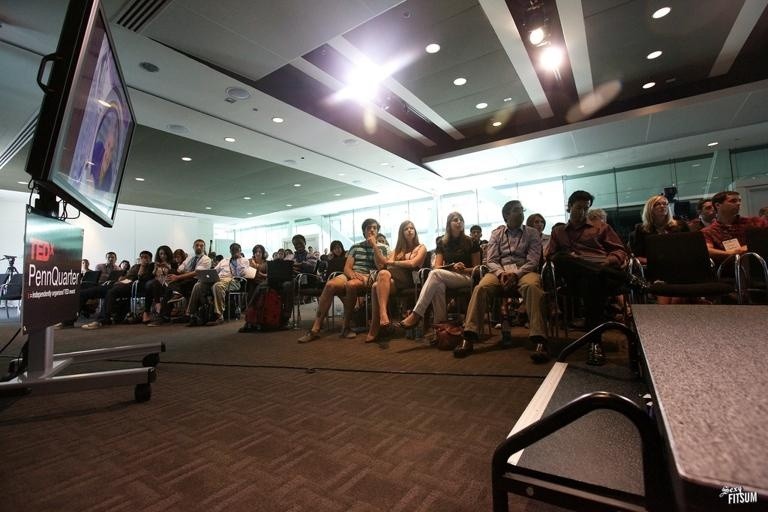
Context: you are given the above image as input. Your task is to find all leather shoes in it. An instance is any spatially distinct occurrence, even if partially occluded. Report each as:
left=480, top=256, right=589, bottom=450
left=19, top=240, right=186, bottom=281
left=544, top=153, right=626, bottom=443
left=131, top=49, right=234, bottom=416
left=624, top=274, right=646, bottom=293
left=366, top=321, right=604, bottom=366
left=206, top=314, right=224, bottom=325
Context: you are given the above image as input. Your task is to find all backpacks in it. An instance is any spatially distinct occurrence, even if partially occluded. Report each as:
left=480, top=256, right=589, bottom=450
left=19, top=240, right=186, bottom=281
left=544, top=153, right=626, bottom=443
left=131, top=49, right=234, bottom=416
left=244, top=286, right=281, bottom=330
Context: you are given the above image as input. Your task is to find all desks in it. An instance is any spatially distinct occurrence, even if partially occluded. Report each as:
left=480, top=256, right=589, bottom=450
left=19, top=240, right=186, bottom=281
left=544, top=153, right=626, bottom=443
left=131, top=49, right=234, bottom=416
left=630, top=305, right=768, bottom=511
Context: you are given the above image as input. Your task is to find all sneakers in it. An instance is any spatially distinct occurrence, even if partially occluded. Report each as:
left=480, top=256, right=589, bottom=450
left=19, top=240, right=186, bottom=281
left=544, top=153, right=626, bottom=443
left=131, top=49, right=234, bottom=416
left=171, top=307, right=184, bottom=316
left=167, top=294, right=184, bottom=303
left=298, top=329, right=319, bottom=342
left=53, top=322, right=74, bottom=329
left=81, top=322, right=102, bottom=329
left=340, top=327, right=355, bottom=338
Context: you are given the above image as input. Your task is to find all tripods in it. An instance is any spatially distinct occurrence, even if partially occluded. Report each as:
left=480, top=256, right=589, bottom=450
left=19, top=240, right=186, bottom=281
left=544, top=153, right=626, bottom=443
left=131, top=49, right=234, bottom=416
left=0, top=254, right=21, bottom=319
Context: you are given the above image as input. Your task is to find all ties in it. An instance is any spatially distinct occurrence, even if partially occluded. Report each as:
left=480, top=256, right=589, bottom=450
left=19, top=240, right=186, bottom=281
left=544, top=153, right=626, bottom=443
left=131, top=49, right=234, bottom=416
left=191, top=257, right=198, bottom=272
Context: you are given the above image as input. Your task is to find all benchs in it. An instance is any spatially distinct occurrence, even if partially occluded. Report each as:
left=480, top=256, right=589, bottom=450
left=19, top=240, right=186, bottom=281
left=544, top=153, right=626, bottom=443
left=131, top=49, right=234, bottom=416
left=491, top=321, right=671, bottom=512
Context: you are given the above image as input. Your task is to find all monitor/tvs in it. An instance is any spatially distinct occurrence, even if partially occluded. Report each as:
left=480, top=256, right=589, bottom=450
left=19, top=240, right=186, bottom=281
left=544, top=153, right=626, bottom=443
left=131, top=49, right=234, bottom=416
left=23, top=0, right=138, bottom=229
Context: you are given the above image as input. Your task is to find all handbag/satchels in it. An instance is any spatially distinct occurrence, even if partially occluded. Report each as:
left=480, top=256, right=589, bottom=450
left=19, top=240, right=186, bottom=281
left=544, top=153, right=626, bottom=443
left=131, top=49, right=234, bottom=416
left=267, top=258, right=293, bottom=290
left=366, top=270, right=378, bottom=288
left=296, top=273, right=325, bottom=287
left=190, top=310, right=206, bottom=326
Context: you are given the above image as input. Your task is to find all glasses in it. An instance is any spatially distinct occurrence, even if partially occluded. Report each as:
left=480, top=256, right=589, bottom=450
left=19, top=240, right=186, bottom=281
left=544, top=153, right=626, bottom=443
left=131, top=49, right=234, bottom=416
left=141, top=254, right=148, bottom=259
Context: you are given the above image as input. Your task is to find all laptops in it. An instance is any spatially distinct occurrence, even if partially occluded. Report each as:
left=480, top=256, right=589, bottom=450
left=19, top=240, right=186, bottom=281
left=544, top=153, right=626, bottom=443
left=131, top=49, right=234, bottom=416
left=195, top=269, right=221, bottom=284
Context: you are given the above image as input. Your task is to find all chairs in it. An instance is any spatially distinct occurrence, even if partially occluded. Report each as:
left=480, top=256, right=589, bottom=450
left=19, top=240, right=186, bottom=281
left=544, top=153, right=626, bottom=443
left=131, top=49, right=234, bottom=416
left=550, top=231, right=636, bottom=347
left=634, top=232, right=743, bottom=304
left=740, top=227, right=768, bottom=304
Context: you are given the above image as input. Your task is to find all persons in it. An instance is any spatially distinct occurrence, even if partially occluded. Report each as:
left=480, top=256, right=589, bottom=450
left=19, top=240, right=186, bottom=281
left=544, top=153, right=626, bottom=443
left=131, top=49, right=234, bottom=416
left=81, top=190, right=768, bottom=365
